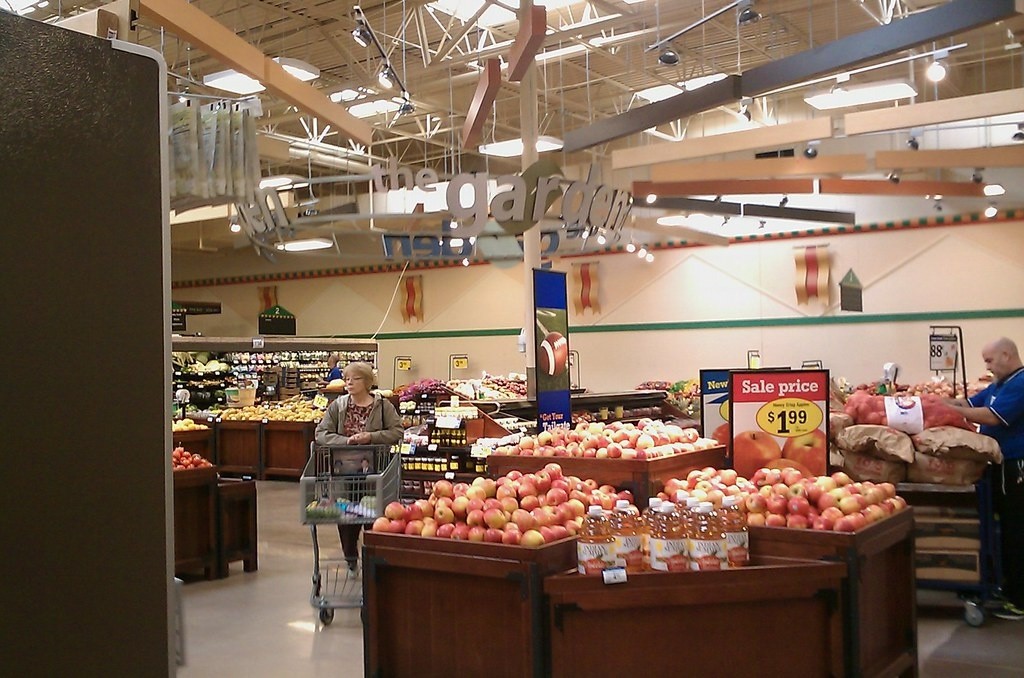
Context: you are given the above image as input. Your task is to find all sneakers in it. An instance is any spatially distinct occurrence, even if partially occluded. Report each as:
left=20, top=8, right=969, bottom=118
left=992, top=602, right=1024, bottom=621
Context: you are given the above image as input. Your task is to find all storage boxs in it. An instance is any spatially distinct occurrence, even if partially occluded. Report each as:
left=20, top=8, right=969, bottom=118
left=268, top=366, right=299, bottom=400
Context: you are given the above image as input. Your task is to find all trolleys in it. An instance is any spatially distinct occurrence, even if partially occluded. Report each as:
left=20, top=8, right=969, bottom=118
left=298, top=437, right=404, bottom=626
left=917, top=465, right=1003, bottom=627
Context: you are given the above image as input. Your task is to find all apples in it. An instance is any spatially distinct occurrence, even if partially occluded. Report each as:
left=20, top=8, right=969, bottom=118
left=173, top=447, right=212, bottom=470
left=372, top=421, right=906, bottom=549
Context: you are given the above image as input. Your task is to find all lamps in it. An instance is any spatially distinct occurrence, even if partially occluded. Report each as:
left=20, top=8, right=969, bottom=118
left=199, top=0, right=1019, bottom=255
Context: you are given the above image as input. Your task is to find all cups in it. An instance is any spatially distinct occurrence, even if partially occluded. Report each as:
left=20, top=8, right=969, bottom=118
left=615, top=406, right=623, bottom=419
left=599, top=407, right=608, bottom=419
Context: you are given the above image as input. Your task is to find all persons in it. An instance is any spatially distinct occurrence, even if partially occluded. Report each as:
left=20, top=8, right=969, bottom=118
left=936, top=337, right=1024, bottom=620
left=318, top=354, right=342, bottom=385
left=357, top=458, right=373, bottom=473
left=333, top=460, right=343, bottom=474
left=315, top=363, right=406, bottom=444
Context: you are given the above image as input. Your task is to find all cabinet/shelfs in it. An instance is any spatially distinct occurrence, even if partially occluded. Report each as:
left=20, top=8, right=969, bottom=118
left=391, top=406, right=487, bottom=501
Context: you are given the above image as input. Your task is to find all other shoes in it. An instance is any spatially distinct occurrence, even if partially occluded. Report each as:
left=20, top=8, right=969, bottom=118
left=348, top=565, right=361, bottom=579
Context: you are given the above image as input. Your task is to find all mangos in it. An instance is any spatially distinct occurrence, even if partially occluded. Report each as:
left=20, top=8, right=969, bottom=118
left=225, top=405, right=324, bottom=422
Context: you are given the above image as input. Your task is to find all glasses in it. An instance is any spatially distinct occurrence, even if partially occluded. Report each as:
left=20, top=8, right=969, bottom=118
left=345, top=375, right=365, bottom=382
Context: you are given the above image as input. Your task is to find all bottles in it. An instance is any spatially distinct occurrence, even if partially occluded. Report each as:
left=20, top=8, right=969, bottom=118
left=609, top=500, right=642, bottom=571
left=440, top=430, right=445, bottom=447
left=462, top=430, right=467, bottom=448
left=436, top=430, right=441, bottom=446
left=681, top=498, right=699, bottom=529
left=675, top=492, right=688, bottom=517
left=451, top=430, right=456, bottom=447
left=650, top=502, right=689, bottom=572
left=641, top=498, right=662, bottom=571
left=576, top=507, right=617, bottom=576
left=717, top=496, right=749, bottom=565
left=688, top=501, right=728, bottom=570
left=430, top=430, right=436, bottom=444
left=456, top=430, right=461, bottom=447
left=445, top=430, right=450, bottom=447
